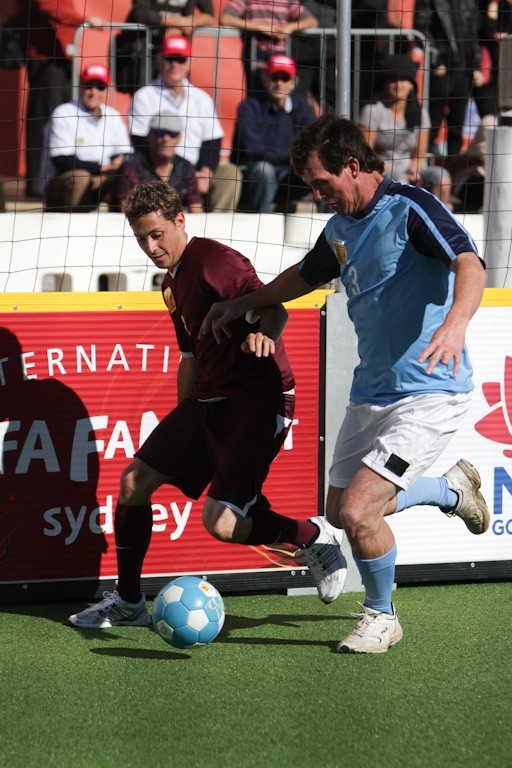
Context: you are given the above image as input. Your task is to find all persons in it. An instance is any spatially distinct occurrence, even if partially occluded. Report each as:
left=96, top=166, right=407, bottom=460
left=0, top=0, right=512, bottom=214
left=68, top=183, right=349, bottom=627
left=200, top=113, right=489, bottom=654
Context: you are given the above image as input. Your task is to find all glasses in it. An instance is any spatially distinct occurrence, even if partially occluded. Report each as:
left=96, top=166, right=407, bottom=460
left=164, top=54, right=188, bottom=64
left=271, top=72, right=292, bottom=84
left=83, top=79, right=107, bottom=91
left=155, top=129, right=180, bottom=138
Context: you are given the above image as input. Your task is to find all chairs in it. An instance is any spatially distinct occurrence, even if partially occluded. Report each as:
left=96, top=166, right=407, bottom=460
left=0, top=0, right=471, bottom=218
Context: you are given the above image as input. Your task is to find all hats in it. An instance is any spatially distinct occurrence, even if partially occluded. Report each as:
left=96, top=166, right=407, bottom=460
left=266, top=54, right=296, bottom=78
left=378, top=55, right=420, bottom=83
left=160, top=35, right=191, bottom=59
left=79, top=65, right=110, bottom=87
left=150, top=111, right=182, bottom=134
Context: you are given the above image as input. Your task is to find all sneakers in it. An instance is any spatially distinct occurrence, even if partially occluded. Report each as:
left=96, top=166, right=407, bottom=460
left=297, top=516, right=347, bottom=604
left=439, top=458, right=491, bottom=535
left=68, top=589, right=150, bottom=627
left=336, top=600, right=403, bottom=653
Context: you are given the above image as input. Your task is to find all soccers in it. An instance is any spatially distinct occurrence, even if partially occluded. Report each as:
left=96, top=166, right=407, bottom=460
left=152, top=576, right=225, bottom=650
